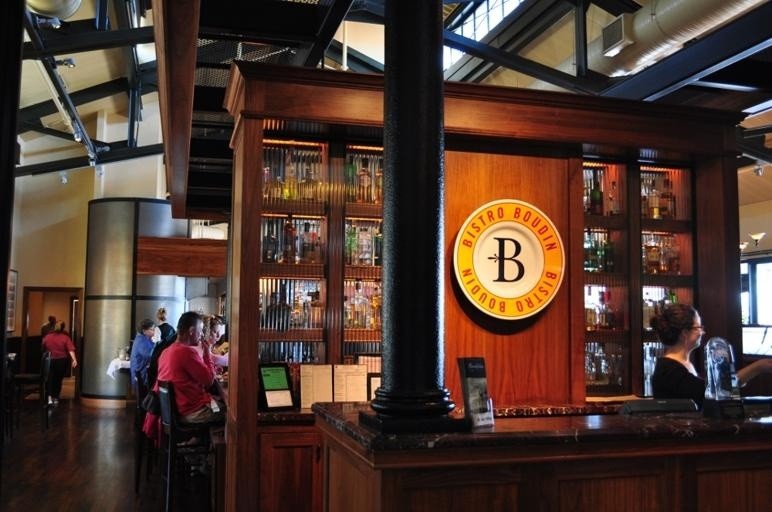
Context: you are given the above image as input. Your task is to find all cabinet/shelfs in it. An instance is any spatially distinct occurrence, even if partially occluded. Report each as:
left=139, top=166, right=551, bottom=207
left=577, top=146, right=700, bottom=396
left=246, top=422, right=324, bottom=509
left=256, top=117, right=382, bottom=408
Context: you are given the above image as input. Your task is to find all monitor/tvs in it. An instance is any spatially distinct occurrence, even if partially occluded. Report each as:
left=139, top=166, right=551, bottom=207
left=620, top=397, right=699, bottom=415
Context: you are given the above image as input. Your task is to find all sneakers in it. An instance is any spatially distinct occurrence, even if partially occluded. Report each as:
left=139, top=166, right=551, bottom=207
left=48, top=399, right=59, bottom=404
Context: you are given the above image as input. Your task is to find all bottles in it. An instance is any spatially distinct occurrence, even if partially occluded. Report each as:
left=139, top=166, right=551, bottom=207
left=262, top=211, right=317, bottom=265
left=374, top=220, right=385, bottom=267
left=265, top=284, right=292, bottom=330
left=644, top=233, right=662, bottom=276
left=345, top=155, right=385, bottom=205
left=648, top=178, right=678, bottom=221
left=308, top=290, right=325, bottom=328
left=299, top=157, right=318, bottom=202
left=348, top=278, right=383, bottom=330
left=590, top=180, right=622, bottom=217
left=598, top=291, right=616, bottom=328
left=583, top=227, right=617, bottom=274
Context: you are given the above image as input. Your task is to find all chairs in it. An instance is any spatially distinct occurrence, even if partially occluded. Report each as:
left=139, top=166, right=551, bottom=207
left=10, top=351, right=53, bottom=436
left=132, top=369, right=214, bottom=510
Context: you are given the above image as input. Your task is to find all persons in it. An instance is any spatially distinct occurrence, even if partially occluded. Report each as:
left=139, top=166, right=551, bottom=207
left=127, top=306, right=228, bottom=481
left=649, top=304, right=772, bottom=401
left=40, top=320, right=78, bottom=407
left=39, top=315, right=56, bottom=338
